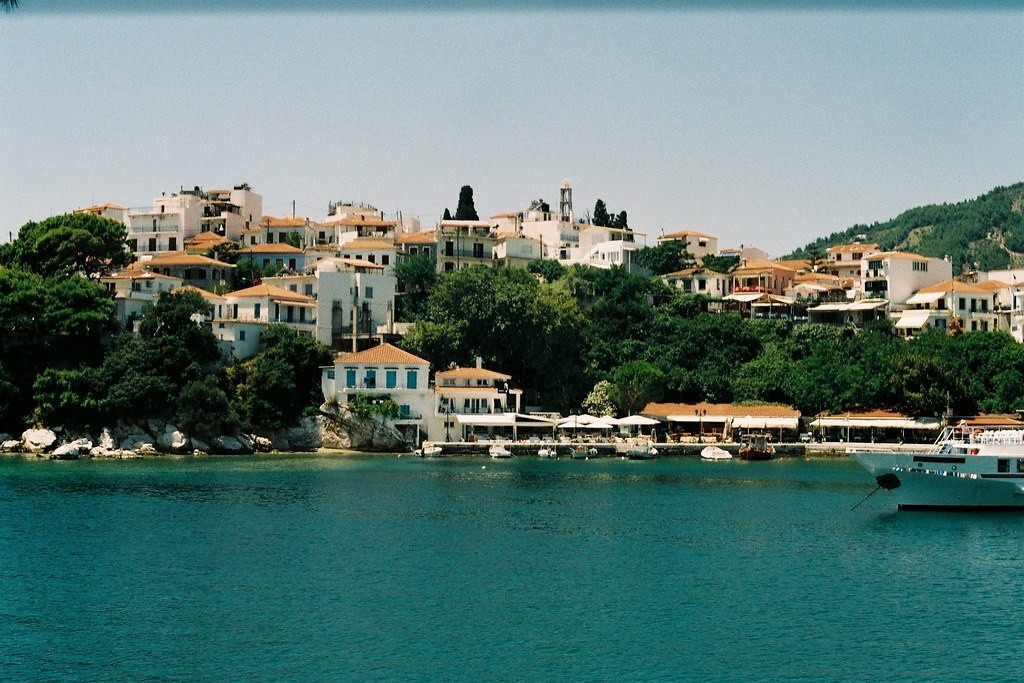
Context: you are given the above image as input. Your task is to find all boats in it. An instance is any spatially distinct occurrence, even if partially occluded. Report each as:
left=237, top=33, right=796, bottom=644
left=844, top=416, right=1024, bottom=513
left=738, top=434, right=774, bottom=461
left=570, top=445, right=598, bottom=459
left=625, top=440, right=660, bottom=458
left=700, top=445, right=734, bottom=459
left=414, top=446, right=443, bottom=457
left=538, top=450, right=557, bottom=458
left=488, top=443, right=512, bottom=458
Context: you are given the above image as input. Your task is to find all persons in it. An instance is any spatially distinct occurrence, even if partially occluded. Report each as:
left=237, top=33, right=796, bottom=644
left=956, top=419, right=983, bottom=444
